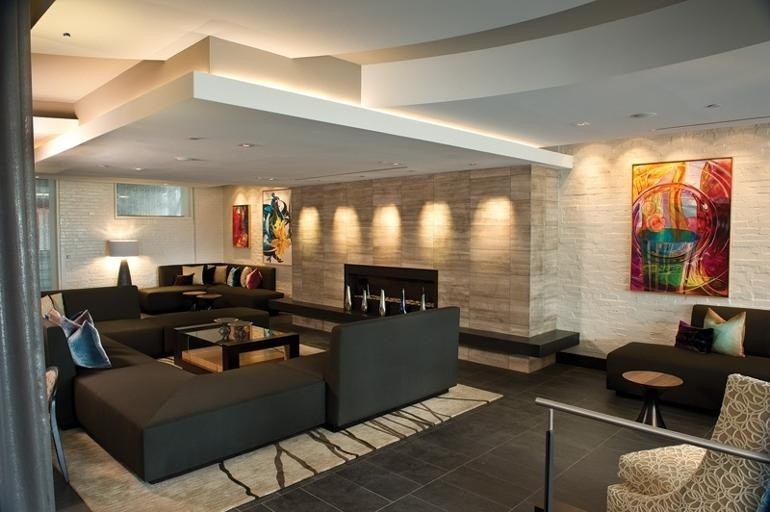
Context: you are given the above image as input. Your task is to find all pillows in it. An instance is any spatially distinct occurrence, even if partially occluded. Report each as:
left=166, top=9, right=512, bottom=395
left=673, top=318, right=715, bottom=355
left=702, top=306, right=747, bottom=360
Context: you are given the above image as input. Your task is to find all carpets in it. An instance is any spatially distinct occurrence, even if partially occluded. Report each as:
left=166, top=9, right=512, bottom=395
left=55, top=328, right=505, bottom=511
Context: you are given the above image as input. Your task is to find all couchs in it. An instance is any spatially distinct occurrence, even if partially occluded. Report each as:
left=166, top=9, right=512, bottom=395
left=42, top=263, right=284, bottom=358
left=603, top=301, right=770, bottom=410
left=44, top=359, right=326, bottom=482
left=279, top=306, right=460, bottom=429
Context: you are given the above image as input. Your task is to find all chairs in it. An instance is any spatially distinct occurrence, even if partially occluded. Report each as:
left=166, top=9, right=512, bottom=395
left=603, top=369, right=769, bottom=512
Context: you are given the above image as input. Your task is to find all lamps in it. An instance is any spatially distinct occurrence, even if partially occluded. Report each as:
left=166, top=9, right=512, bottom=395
left=106, top=240, right=142, bottom=285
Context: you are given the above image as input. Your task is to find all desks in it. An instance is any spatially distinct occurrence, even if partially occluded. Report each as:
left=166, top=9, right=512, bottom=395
left=621, top=370, right=684, bottom=430
left=173, top=320, right=301, bottom=374
